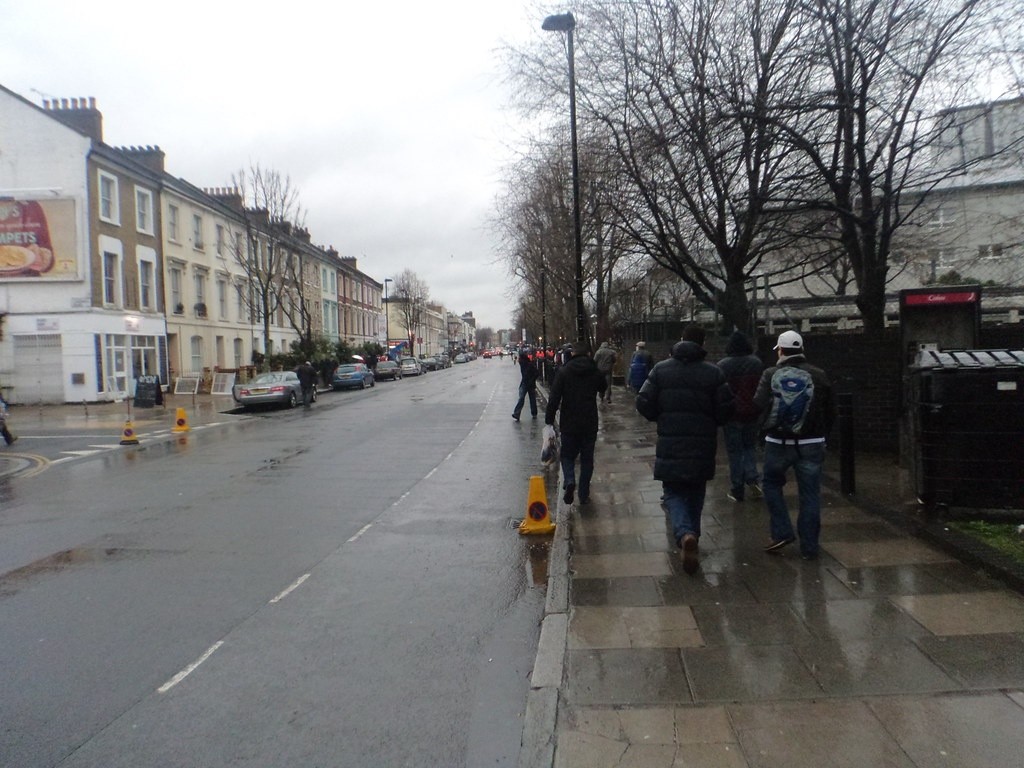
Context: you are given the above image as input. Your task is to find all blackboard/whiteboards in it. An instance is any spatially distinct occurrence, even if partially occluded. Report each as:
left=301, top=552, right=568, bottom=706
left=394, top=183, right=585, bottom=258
left=133, top=374, right=163, bottom=408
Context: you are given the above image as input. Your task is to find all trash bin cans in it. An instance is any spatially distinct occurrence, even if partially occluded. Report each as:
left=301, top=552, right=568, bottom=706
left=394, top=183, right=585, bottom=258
left=909, top=343, right=1024, bottom=514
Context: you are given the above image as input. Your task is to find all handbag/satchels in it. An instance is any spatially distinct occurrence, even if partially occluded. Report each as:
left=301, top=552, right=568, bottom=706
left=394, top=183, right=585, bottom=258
left=540, top=424, right=557, bottom=465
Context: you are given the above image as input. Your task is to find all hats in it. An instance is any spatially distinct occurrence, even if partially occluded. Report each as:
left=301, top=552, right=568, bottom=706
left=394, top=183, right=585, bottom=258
left=634, top=341, right=645, bottom=347
left=772, top=331, right=803, bottom=351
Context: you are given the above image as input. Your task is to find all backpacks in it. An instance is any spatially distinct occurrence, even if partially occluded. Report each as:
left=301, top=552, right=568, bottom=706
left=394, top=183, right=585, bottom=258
left=761, top=364, right=814, bottom=438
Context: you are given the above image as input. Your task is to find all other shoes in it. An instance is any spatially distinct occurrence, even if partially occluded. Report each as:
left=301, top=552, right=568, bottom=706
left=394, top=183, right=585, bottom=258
left=764, top=533, right=795, bottom=550
left=677, top=532, right=698, bottom=547
left=727, top=491, right=743, bottom=500
left=563, top=482, right=575, bottom=505
left=533, top=415, right=537, bottom=418
left=600, top=398, right=604, bottom=403
left=748, top=479, right=762, bottom=497
left=580, top=496, right=591, bottom=504
left=512, top=414, right=520, bottom=419
left=607, top=398, right=611, bottom=404
left=7, top=436, right=18, bottom=445
left=802, top=550, right=819, bottom=559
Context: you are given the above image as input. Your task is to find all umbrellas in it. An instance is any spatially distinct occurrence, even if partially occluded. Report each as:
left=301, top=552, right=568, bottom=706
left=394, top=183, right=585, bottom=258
left=351, top=355, right=363, bottom=360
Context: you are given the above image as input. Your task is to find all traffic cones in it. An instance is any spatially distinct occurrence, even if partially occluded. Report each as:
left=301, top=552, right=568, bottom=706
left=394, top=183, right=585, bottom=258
left=118, top=418, right=138, bottom=445
left=518, top=475, right=554, bottom=535
left=172, top=408, right=190, bottom=432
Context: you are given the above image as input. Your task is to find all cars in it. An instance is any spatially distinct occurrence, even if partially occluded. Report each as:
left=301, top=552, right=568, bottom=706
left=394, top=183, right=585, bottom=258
left=374, top=361, right=402, bottom=380
left=482, top=348, right=508, bottom=358
left=333, top=363, right=375, bottom=390
left=454, top=351, right=478, bottom=363
left=401, top=357, right=422, bottom=375
left=232, top=370, right=316, bottom=407
left=422, top=355, right=451, bottom=372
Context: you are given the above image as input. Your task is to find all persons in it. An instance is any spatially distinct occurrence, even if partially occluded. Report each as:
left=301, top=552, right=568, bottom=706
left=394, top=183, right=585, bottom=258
left=754, top=330, right=829, bottom=558
left=593, top=343, right=617, bottom=403
left=297, top=361, right=318, bottom=408
left=511, top=349, right=540, bottom=420
left=630, top=341, right=654, bottom=370
left=545, top=343, right=608, bottom=505
left=635, top=325, right=736, bottom=578
left=0, top=391, right=18, bottom=445
left=536, top=345, right=571, bottom=392
left=627, top=355, right=649, bottom=411
left=716, top=331, right=766, bottom=501
left=313, top=358, right=339, bottom=388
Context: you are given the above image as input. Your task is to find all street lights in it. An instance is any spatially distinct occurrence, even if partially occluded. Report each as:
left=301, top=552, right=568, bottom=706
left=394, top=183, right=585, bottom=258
left=417, top=297, right=421, bottom=356
left=541, top=11, right=586, bottom=354
left=385, top=279, right=394, bottom=356
